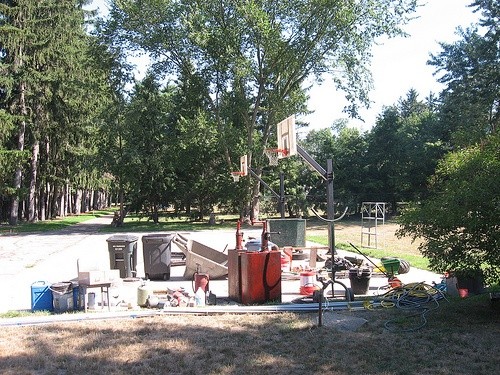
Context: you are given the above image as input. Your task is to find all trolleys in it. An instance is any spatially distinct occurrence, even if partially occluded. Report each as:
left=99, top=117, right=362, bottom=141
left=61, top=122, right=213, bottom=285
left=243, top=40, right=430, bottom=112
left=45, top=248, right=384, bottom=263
left=347, top=241, right=402, bottom=288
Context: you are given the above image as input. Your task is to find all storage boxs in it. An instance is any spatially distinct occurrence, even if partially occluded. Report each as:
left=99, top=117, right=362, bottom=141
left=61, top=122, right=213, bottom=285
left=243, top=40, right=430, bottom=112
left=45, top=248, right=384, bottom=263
left=77, top=258, right=105, bottom=286
left=349, top=269, right=373, bottom=295
left=30, top=284, right=53, bottom=312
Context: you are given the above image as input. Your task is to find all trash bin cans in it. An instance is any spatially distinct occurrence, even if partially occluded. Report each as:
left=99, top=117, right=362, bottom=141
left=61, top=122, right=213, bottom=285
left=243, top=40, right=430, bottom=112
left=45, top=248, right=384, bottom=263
left=141, top=234, right=173, bottom=280
left=106, top=235, right=138, bottom=278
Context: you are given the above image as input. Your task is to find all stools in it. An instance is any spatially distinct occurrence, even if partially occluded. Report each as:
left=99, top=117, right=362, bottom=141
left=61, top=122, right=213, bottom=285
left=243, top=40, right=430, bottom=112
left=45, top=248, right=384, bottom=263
left=78, top=284, right=112, bottom=312
